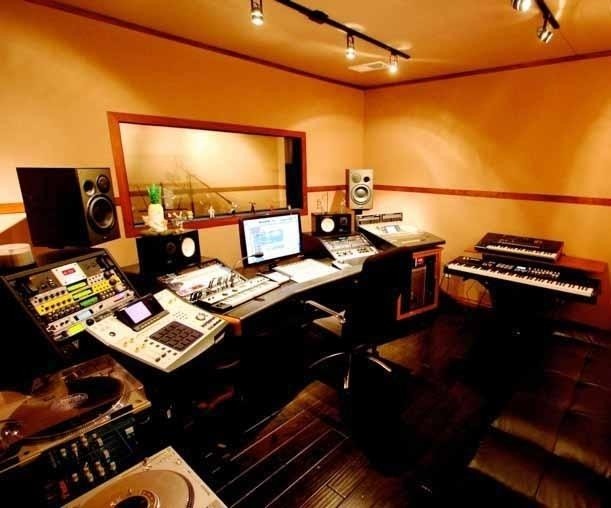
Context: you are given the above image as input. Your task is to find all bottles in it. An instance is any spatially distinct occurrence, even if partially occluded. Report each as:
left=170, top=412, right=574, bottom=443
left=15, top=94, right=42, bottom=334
left=148, top=203, right=164, bottom=232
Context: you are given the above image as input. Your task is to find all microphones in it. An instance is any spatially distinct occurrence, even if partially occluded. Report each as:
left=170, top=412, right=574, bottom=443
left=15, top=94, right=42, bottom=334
left=230, top=252, right=263, bottom=284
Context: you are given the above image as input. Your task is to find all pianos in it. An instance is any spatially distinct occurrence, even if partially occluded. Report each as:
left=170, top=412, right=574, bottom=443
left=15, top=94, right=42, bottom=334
left=444, top=255, right=601, bottom=304
left=475, top=232, right=565, bottom=262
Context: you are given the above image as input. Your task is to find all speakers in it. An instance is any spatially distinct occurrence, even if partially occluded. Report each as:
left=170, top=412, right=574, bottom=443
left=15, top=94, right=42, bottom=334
left=16, top=168, right=121, bottom=248
left=311, top=213, right=351, bottom=236
left=136, top=228, right=201, bottom=275
left=346, top=168, right=374, bottom=210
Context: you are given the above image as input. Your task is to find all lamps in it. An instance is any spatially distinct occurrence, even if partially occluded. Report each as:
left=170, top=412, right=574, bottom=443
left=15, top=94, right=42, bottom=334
left=346, top=34, right=357, bottom=60
left=510, top=0, right=531, bottom=11
left=537, top=15, right=554, bottom=43
left=388, top=51, right=399, bottom=74
left=250, top=0, right=265, bottom=26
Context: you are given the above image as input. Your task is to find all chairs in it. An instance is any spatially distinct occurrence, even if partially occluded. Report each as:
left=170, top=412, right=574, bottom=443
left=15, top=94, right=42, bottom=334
left=301, top=243, right=413, bottom=393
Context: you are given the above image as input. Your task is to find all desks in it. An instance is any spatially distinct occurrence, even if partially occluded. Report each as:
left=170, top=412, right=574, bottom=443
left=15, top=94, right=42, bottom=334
left=207, top=238, right=444, bottom=395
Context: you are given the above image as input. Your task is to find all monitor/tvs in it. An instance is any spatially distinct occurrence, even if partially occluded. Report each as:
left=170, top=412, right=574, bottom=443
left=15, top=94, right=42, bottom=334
left=238, top=211, right=304, bottom=275
